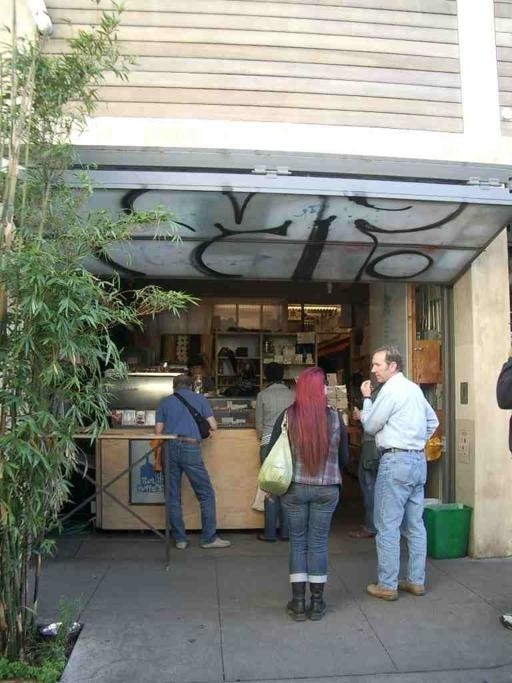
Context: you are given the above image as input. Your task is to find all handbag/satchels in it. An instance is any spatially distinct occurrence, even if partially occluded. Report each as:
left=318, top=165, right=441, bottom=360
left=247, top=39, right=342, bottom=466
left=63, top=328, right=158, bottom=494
left=255, top=407, right=295, bottom=498
left=197, top=416, right=211, bottom=440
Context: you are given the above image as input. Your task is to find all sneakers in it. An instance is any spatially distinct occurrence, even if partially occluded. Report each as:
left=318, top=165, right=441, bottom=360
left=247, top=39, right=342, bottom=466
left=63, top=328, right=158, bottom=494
left=256, top=531, right=290, bottom=543
left=347, top=527, right=375, bottom=539
left=175, top=541, right=188, bottom=548
left=366, top=582, right=399, bottom=601
left=398, top=579, right=425, bottom=596
left=200, top=535, right=232, bottom=548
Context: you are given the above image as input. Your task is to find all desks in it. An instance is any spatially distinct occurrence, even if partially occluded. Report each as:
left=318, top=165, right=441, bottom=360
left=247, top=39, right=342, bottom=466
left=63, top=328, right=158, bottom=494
left=49, top=427, right=181, bottom=572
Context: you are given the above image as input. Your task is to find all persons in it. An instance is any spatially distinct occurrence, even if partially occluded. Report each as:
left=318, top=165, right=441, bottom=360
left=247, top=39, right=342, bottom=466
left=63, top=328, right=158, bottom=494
left=496, top=347, right=512, bottom=632
left=150, top=375, right=231, bottom=549
left=359, top=343, right=440, bottom=602
left=255, top=362, right=296, bottom=543
left=264, top=365, right=350, bottom=622
left=347, top=381, right=383, bottom=539
left=186, top=357, right=219, bottom=398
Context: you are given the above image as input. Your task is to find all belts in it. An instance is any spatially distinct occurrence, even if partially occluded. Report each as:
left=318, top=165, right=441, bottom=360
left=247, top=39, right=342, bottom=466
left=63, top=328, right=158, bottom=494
left=380, top=446, right=425, bottom=456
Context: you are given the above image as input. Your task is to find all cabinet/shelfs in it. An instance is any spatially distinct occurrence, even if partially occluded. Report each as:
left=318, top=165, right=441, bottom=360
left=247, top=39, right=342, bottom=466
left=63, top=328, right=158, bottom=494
left=212, top=328, right=321, bottom=396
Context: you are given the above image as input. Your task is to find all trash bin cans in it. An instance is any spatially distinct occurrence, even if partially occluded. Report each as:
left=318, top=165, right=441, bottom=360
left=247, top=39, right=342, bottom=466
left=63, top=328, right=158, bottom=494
left=424, top=503, right=473, bottom=558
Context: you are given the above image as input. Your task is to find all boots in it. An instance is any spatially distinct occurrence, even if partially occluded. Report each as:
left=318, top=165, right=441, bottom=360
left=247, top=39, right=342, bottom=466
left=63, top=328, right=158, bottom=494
left=309, top=582, right=329, bottom=621
left=285, top=582, right=308, bottom=622
